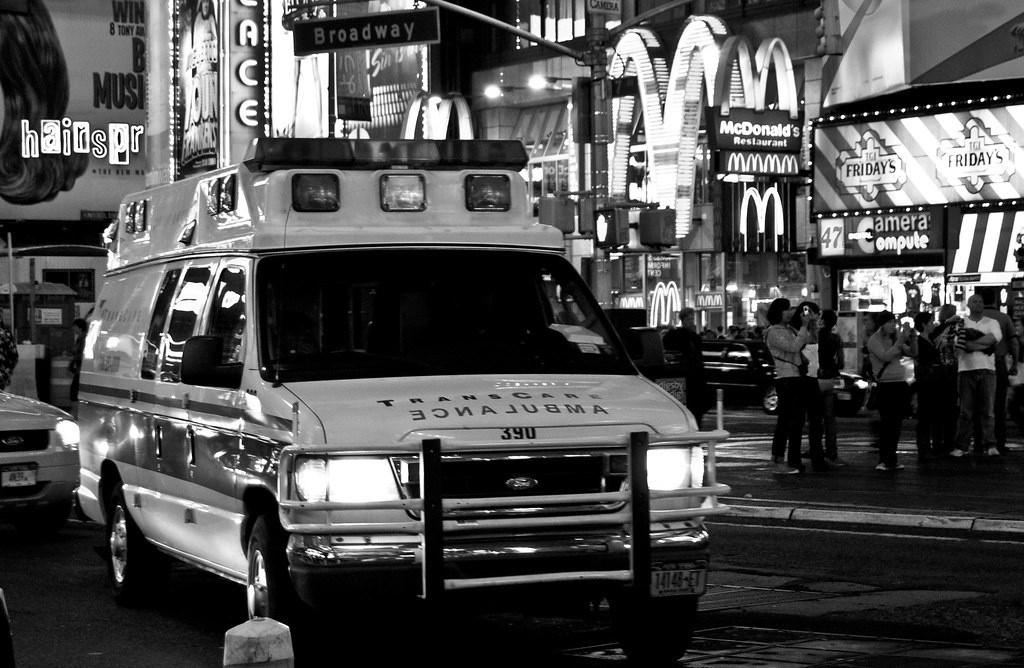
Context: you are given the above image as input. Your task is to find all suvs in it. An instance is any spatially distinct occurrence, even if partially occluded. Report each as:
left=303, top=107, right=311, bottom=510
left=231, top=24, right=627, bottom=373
left=700, top=338, right=870, bottom=418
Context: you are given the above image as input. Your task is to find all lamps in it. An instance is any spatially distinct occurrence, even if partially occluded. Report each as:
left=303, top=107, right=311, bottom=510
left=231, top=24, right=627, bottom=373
left=485, top=82, right=528, bottom=101
left=526, top=75, right=572, bottom=90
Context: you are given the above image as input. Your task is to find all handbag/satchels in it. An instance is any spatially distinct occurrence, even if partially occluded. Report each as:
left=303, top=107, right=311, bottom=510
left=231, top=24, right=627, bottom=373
left=798, top=360, right=809, bottom=376
left=864, top=374, right=877, bottom=411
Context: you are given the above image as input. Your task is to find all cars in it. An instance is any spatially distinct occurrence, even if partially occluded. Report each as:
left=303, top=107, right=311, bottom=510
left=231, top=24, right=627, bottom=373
left=1, top=387, right=83, bottom=525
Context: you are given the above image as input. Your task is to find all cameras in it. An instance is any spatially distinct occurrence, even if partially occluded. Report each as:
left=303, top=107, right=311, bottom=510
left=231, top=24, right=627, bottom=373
left=803, top=306, right=809, bottom=316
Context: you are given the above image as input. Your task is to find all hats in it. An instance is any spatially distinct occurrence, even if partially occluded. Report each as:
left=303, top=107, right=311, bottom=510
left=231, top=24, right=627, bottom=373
left=879, top=310, right=895, bottom=325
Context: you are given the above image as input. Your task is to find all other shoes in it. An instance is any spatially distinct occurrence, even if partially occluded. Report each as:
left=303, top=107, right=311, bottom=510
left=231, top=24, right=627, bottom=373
left=824, top=456, right=848, bottom=468
left=895, top=463, right=907, bottom=469
left=986, top=445, right=1000, bottom=456
left=948, top=449, right=972, bottom=457
left=875, top=462, right=887, bottom=472
left=774, top=463, right=799, bottom=476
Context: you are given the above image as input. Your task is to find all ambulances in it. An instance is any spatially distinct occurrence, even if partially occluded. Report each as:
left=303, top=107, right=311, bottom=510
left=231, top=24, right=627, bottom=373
left=70, top=134, right=734, bottom=668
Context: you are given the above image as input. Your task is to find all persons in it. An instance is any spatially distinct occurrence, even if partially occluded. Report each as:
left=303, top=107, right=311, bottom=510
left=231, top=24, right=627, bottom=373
left=70, top=318, right=87, bottom=420
left=671, top=294, right=1024, bottom=475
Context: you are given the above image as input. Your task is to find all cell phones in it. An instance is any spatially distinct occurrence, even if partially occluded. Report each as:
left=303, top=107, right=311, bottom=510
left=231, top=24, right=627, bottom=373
left=903, top=322, right=909, bottom=330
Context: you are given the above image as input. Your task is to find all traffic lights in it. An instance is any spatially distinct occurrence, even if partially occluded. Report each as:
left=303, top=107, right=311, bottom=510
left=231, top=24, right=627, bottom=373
left=637, top=205, right=677, bottom=249
left=592, top=207, right=629, bottom=249
